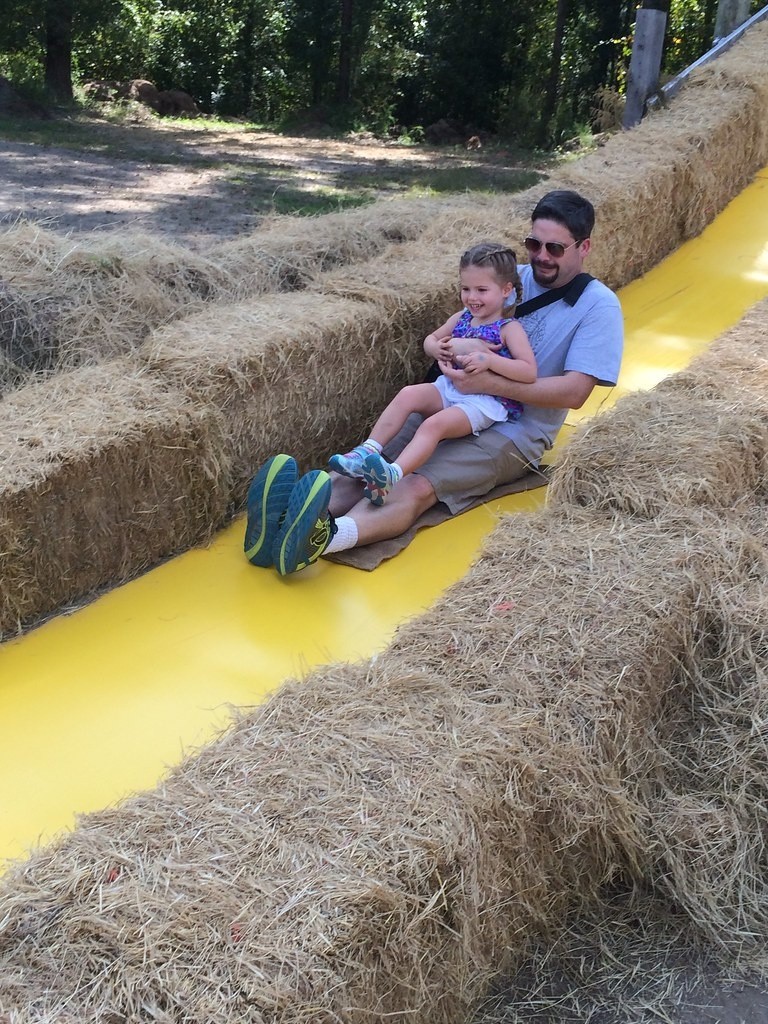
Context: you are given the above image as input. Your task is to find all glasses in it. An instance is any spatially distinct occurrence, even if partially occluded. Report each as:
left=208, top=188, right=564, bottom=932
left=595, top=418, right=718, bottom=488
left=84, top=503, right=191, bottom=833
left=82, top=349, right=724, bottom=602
left=523, top=232, right=580, bottom=258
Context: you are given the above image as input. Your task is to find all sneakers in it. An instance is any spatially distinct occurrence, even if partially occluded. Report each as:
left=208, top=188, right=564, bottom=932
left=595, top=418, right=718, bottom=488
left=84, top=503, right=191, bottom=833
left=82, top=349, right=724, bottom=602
left=243, top=454, right=298, bottom=568
left=361, top=453, right=398, bottom=506
left=271, top=470, right=338, bottom=577
left=328, top=447, right=369, bottom=483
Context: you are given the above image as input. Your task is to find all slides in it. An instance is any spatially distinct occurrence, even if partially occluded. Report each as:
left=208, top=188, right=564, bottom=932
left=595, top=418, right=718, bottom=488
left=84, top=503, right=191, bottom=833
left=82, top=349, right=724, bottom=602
left=3, top=18, right=768, bottom=1024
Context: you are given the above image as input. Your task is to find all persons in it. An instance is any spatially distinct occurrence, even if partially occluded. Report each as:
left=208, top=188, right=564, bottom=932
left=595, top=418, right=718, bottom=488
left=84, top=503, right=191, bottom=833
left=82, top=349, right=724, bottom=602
left=243, top=191, right=623, bottom=576
left=328, top=242, right=536, bottom=506
